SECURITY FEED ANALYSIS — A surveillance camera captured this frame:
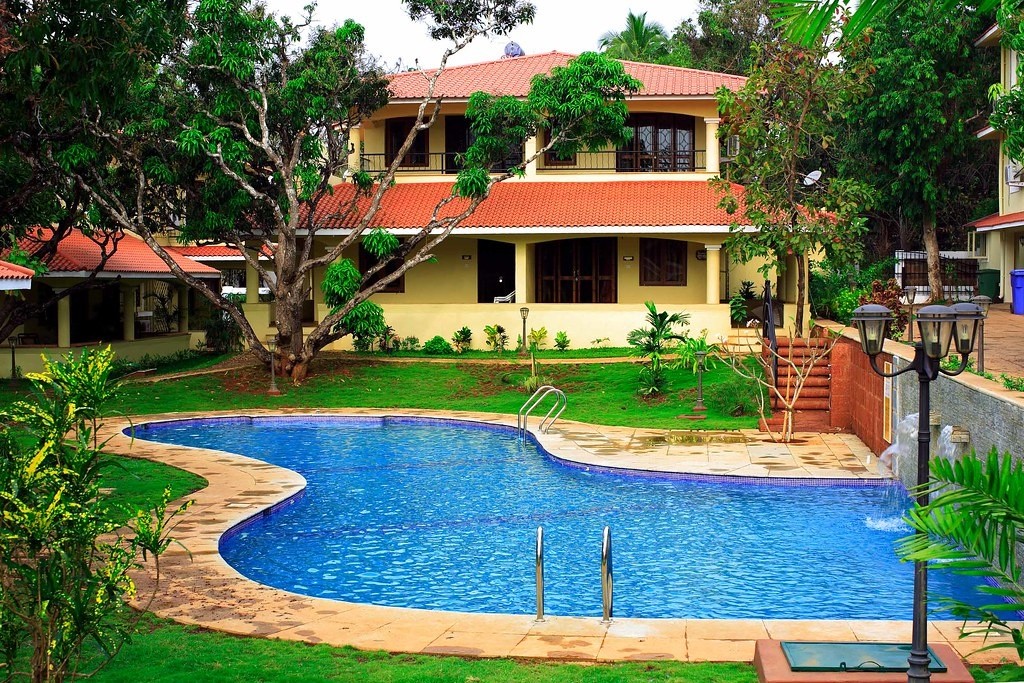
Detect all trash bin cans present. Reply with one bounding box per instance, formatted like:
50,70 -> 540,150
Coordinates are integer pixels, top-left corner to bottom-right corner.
1009,269 -> 1024,314
976,268 -> 1001,302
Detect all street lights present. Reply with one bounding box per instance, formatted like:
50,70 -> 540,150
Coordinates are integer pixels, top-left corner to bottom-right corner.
519,307 -> 529,356
848,298 -> 986,683
693,350 -> 707,410
267,338 -> 281,395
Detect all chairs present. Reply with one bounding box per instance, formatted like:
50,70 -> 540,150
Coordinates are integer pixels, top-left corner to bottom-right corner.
17,333 -> 40,345
494,289 -> 515,304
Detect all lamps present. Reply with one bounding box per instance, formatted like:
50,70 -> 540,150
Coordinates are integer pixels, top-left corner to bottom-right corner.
8,336 -> 21,389
266,339 -> 280,396
903,286 -> 918,347
693,350 -> 708,413
519,306 -> 531,356
972,293 -> 992,377
